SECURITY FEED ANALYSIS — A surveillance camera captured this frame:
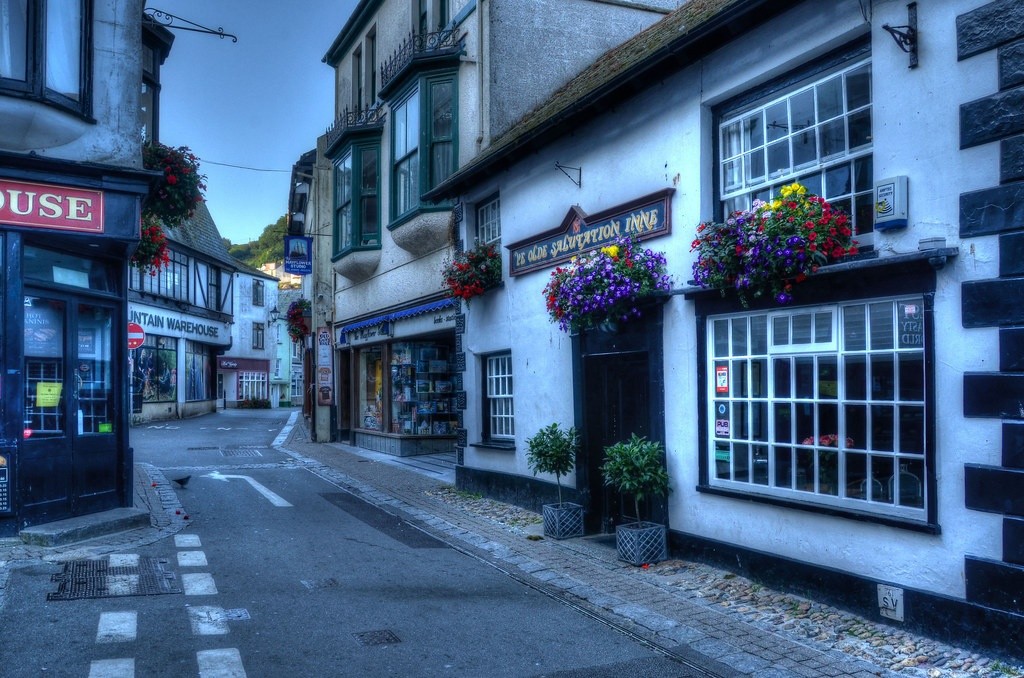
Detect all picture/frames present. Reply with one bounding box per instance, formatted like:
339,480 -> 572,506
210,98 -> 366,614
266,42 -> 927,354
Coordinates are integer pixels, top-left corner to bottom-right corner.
741,401 -> 762,439
741,362 -> 761,398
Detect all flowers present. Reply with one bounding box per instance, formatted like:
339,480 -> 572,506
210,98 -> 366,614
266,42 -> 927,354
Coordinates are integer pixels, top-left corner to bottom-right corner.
802,434 -> 854,470
689,182 -> 861,304
286,299 -> 312,343
541,235 -> 673,333
144,142 -> 207,229
129,219 -> 170,276
442,237 -> 502,300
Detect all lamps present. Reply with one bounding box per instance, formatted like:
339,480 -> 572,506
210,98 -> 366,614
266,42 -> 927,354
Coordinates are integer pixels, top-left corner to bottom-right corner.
268,305 -> 280,328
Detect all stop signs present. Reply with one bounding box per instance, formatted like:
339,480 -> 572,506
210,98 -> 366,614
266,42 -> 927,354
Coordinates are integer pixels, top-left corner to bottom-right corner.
128,321 -> 145,351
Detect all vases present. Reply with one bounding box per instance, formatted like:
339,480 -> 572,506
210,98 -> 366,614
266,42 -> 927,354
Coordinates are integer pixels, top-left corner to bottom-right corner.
302,311 -> 311,330
810,464 -> 835,495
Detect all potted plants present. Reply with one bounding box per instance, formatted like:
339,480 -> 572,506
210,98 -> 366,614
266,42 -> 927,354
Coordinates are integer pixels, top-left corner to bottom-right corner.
523,422 -> 585,540
597,432 -> 674,566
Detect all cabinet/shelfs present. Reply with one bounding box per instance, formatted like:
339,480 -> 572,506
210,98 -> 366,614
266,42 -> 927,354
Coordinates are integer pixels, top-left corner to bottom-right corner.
391,344 -> 457,435
